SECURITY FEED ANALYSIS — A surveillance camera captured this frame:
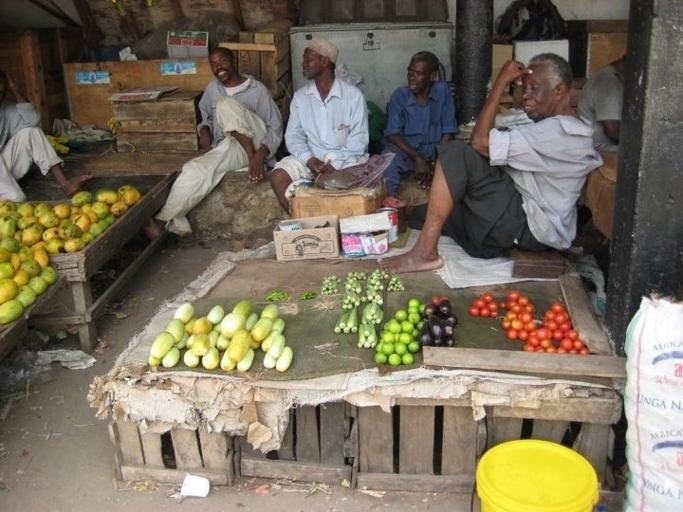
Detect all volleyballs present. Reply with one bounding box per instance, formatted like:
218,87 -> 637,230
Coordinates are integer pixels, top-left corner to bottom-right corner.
419,299 -> 457,346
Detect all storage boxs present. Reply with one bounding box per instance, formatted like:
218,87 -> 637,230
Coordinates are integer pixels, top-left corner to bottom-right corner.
290,176 -> 390,218
580,151 -> 620,241
113,89 -> 207,154
585,19 -> 629,78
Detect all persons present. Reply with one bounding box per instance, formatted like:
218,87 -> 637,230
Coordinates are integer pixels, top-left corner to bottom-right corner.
269,36 -> 371,219
380,51 -> 461,199
576,51 -> 626,154
0,69 -> 94,205
375,51 -> 604,275
142,46 -> 285,240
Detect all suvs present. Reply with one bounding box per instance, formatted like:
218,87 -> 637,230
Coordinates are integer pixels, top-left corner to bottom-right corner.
470,439 -> 605,512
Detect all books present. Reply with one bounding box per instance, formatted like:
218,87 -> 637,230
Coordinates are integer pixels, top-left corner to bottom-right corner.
290,176 -> 390,218
580,151 -> 620,241
113,89 -> 207,154
585,19 -> 629,78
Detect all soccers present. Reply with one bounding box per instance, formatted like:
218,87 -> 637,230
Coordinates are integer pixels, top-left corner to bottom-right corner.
374,298 -> 426,366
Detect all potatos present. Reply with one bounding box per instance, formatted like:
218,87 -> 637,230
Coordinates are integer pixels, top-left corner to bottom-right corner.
148,301 -> 293,372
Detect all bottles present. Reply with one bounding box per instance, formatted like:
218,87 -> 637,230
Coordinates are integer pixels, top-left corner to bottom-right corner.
303,37 -> 339,65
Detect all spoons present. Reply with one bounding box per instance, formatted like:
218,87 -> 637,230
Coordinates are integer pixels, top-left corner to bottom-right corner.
432,295 -> 448,305
469,290 -> 589,354
